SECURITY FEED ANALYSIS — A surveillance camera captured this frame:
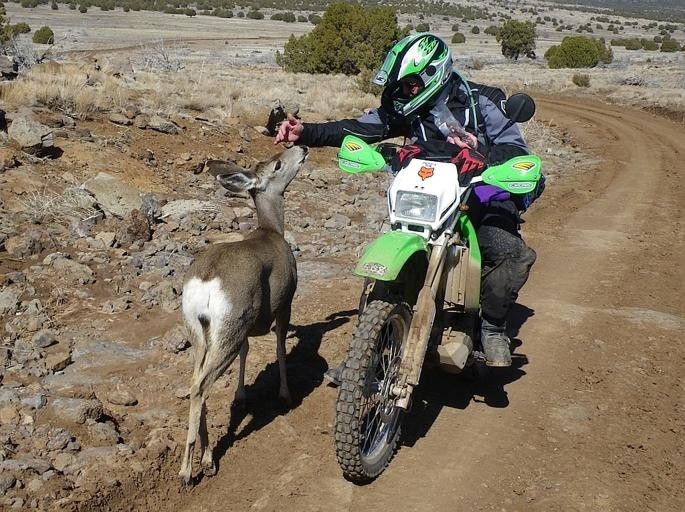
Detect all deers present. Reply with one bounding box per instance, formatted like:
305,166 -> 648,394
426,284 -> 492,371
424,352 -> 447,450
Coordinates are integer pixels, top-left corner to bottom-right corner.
178,145 -> 310,492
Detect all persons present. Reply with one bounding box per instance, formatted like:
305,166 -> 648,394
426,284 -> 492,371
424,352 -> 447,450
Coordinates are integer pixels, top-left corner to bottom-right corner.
272,33 -> 543,386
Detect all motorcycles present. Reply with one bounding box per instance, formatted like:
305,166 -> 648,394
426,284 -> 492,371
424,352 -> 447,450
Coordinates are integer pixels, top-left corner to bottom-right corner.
334,92 -> 538,483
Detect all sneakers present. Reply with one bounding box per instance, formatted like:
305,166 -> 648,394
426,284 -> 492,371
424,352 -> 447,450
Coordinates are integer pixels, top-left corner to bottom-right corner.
479,326 -> 514,368
322,360 -> 349,384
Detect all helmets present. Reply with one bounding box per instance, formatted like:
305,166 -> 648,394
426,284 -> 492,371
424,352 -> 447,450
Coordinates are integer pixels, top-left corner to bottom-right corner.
372,33 -> 461,119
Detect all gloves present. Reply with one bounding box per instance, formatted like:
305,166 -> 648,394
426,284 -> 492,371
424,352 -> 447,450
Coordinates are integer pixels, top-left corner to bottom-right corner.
451,144 -> 491,188
388,140 -> 428,174
